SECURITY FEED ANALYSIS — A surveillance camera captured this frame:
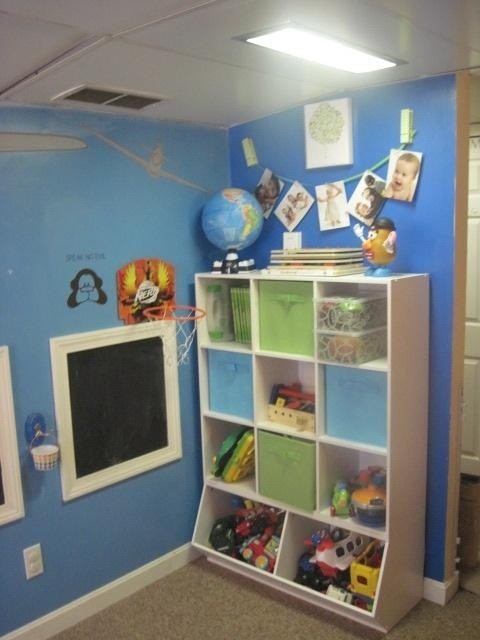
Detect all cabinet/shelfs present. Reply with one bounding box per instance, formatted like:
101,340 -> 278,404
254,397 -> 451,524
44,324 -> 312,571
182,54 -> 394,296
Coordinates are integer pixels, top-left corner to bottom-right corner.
187,268 -> 429,633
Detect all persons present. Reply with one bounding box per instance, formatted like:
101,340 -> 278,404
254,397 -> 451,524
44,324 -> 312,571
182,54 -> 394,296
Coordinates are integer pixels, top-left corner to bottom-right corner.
255,152 -> 420,226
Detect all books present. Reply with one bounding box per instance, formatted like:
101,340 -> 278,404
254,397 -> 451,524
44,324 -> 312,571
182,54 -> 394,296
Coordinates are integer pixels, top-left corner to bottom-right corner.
267,248 -> 365,277
230,283 -> 250,345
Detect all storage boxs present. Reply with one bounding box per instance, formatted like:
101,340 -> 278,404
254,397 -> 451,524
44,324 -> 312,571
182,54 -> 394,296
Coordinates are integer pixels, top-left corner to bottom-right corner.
317,293 -> 384,365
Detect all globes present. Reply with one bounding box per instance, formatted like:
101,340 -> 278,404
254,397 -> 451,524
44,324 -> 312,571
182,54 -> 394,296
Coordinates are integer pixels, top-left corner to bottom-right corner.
200,187 -> 266,274
